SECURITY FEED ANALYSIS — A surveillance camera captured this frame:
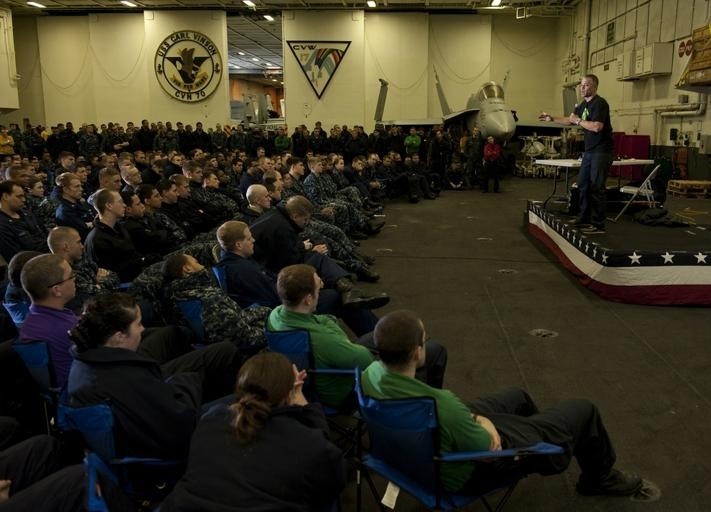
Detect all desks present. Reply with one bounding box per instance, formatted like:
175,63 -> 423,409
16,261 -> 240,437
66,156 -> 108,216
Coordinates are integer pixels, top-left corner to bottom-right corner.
535,157 -> 655,228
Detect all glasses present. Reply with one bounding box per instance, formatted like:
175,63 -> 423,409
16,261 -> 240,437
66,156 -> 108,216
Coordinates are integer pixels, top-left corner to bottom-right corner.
48,272 -> 77,288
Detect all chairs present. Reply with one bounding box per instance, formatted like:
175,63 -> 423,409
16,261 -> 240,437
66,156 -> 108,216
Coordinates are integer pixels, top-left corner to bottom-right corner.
0,238 -> 565,512
607,164 -> 661,222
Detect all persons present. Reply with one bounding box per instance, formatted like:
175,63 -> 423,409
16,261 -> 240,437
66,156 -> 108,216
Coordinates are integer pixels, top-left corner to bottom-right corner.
153,351 -> 343,510
65,291 -> 247,459
1,434 -> 137,510
359,310 -> 644,496
0,119 -> 499,450
539,74 -> 616,235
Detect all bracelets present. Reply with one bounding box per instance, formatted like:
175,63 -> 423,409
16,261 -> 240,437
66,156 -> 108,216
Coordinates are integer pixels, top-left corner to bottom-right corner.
470,410 -> 480,424
577,119 -> 583,125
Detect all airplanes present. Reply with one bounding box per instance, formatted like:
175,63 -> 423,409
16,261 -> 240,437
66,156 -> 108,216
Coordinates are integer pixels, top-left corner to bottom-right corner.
374,64 -> 587,146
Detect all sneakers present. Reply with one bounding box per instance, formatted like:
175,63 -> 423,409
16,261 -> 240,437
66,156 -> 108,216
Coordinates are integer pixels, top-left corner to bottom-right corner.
581,224 -> 606,234
569,219 -> 592,226
576,468 -> 641,496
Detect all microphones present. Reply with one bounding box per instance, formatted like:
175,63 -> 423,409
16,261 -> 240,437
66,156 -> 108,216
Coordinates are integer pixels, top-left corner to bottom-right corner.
575,104 -> 579,115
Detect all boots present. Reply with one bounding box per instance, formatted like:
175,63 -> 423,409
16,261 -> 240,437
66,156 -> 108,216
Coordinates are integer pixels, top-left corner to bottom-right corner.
351,201 -> 386,238
357,254 -> 381,280
336,277 -> 389,310
